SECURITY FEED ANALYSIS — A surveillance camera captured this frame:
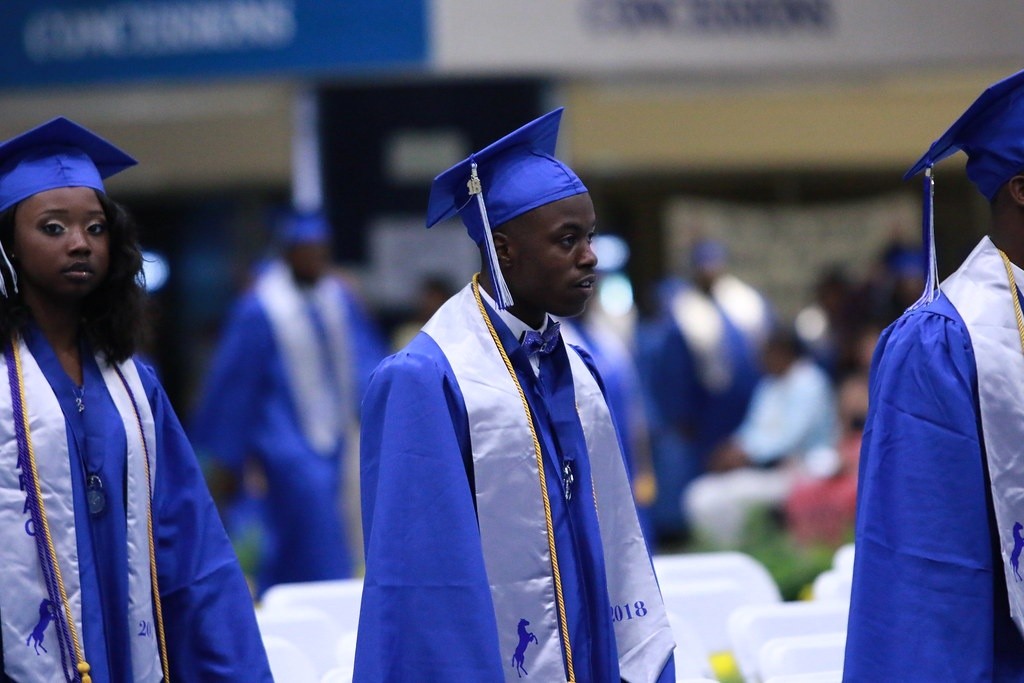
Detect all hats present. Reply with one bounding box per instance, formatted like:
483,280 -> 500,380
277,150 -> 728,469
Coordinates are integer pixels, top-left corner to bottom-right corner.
0,116 -> 139,298
425,106 -> 589,310
903,70 -> 1024,314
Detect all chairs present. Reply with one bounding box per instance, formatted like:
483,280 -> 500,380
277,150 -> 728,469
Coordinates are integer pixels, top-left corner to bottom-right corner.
230,531 -> 861,681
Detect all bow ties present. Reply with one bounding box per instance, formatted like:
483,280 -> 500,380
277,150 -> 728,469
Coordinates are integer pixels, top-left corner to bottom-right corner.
521,322 -> 560,355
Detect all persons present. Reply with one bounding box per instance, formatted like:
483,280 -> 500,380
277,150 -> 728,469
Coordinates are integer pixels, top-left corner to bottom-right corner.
843,71 -> 1024,683
0,117 -> 275,683
186,211 -> 454,601
352,107 -> 677,683
564,234 -> 925,599
